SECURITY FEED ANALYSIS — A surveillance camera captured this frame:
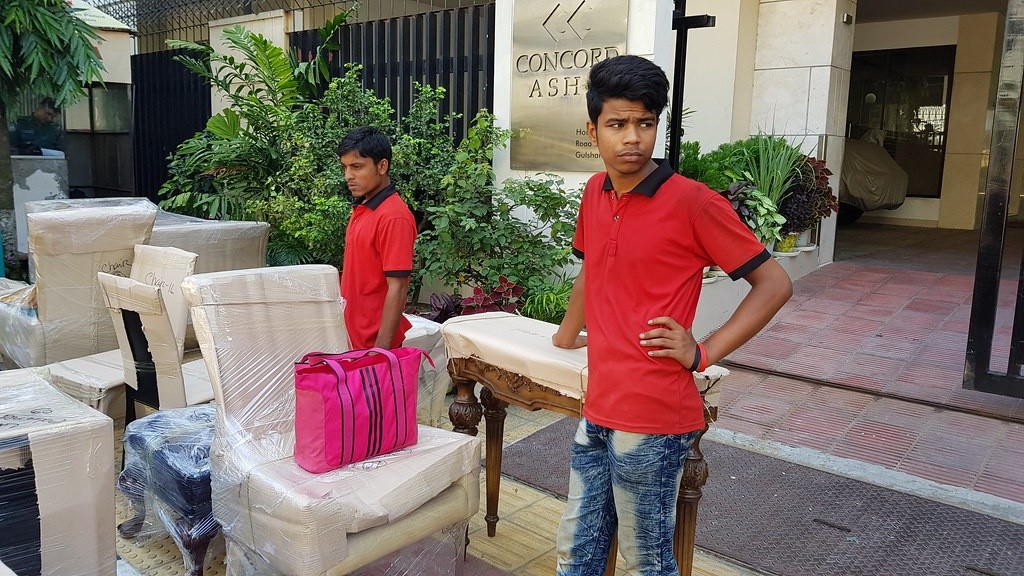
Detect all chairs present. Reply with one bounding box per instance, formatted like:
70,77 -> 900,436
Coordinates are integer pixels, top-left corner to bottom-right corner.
2,202 -> 481,576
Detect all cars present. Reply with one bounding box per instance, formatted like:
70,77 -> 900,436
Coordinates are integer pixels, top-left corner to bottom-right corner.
837,138 -> 910,231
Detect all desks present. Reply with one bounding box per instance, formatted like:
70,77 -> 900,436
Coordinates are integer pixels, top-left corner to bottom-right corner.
440,311 -> 731,576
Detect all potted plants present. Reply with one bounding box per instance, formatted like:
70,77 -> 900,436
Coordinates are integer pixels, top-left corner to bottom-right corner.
725,123 -> 836,257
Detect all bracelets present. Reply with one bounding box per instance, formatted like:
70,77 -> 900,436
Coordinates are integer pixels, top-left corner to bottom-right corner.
688,343 -> 707,373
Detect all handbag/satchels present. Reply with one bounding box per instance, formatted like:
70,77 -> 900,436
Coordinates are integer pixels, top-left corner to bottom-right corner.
293,345 -> 436,474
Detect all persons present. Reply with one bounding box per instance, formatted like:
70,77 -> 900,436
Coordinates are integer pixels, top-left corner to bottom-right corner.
552,54 -> 793,576
336,127 -> 418,358
